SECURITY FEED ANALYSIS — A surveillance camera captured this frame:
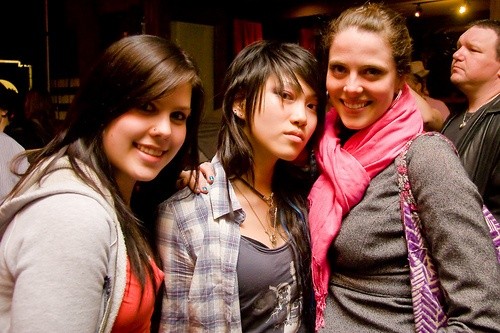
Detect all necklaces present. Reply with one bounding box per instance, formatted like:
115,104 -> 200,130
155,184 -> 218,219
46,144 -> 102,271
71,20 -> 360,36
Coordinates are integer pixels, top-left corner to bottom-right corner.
239,176 -> 280,228
233,182 -> 278,248
459,90 -> 500,128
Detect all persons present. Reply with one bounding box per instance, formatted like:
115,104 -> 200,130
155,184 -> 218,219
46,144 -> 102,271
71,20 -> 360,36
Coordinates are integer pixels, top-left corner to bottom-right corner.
0,0 -> 500,333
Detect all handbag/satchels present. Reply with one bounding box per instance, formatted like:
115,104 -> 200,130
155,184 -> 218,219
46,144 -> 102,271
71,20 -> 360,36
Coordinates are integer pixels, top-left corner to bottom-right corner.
393,130 -> 500,333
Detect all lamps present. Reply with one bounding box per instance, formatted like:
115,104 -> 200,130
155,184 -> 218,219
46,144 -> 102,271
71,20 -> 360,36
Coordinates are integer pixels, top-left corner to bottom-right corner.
414,4 -> 423,17
